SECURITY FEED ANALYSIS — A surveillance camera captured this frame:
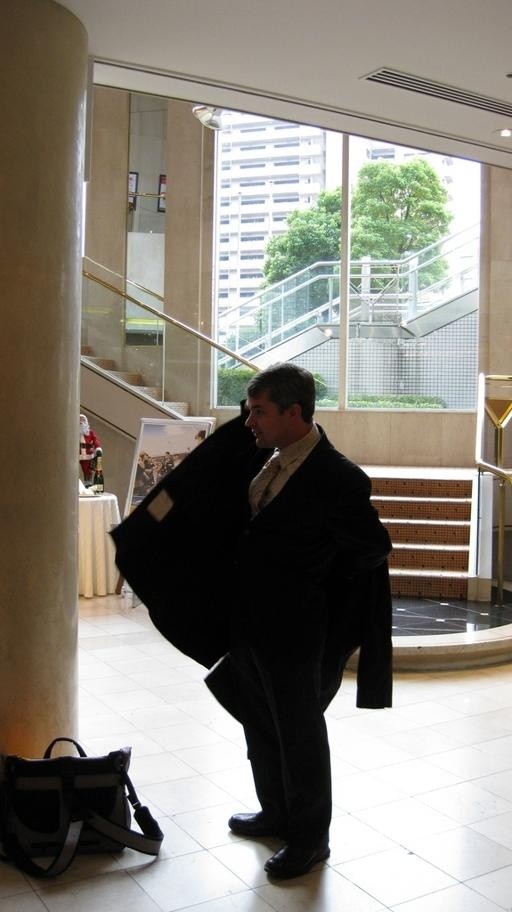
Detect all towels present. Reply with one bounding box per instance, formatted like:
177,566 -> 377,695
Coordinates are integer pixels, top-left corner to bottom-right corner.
78,479 -> 98,495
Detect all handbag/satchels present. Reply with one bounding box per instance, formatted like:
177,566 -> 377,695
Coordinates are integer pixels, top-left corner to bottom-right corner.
0,737 -> 164,880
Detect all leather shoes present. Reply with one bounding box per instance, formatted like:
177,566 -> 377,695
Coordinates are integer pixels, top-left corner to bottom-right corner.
228,810 -> 331,880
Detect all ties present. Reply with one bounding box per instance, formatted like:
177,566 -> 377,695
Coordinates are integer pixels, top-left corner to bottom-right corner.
248,458 -> 281,513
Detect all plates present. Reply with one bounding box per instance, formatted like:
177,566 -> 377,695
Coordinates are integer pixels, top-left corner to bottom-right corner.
79,495 -> 101,498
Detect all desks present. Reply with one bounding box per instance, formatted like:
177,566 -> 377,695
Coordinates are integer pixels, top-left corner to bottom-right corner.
78,491 -> 122,598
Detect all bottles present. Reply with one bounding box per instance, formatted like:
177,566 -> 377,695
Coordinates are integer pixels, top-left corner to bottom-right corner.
94,457 -> 104,493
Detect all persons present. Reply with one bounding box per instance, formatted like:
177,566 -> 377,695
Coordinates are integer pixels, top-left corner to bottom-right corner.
78,412 -> 102,487
207,360 -> 375,882
131,430 -> 205,507
